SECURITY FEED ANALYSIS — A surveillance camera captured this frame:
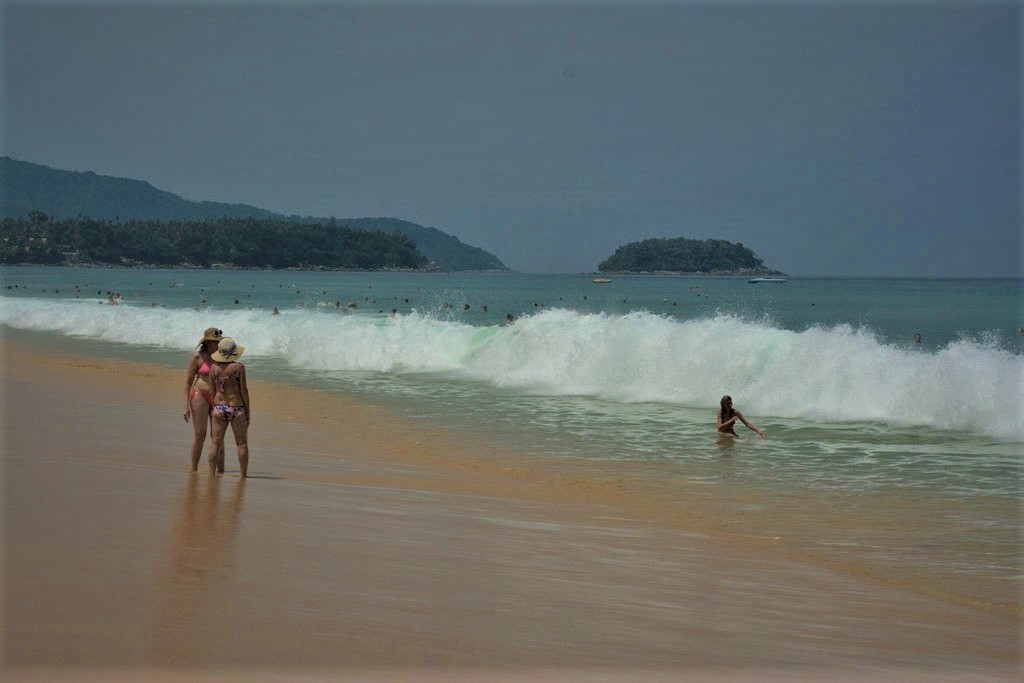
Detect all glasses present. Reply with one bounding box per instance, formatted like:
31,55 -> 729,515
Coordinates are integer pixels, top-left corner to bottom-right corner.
211,330 -> 223,336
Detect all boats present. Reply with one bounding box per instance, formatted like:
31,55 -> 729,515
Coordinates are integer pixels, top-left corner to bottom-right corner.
749,277 -> 787,284
593,279 -> 612,284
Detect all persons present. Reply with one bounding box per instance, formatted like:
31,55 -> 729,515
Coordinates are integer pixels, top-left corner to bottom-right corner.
208,337 -> 250,477
914,333 -> 921,344
183,327 -> 229,474
1,280 -> 679,322
717,395 -> 768,439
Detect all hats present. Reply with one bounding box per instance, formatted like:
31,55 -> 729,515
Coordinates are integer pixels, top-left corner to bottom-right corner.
210,337 -> 244,363
196,327 -> 223,347
